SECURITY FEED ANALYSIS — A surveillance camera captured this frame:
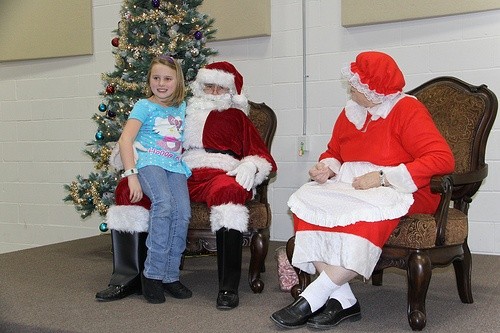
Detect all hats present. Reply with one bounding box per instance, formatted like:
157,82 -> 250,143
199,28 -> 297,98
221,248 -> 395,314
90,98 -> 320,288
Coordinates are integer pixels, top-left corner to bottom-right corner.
340,51 -> 405,104
195,61 -> 244,95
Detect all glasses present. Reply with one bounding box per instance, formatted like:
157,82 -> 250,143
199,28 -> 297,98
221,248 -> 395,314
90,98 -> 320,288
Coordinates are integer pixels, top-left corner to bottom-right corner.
203,88 -> 228,95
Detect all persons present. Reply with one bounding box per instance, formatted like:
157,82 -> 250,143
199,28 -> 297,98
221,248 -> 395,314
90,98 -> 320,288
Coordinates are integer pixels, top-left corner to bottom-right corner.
270,49 -> 456,330
119,55 -> 192,303
93,60 -> 277,309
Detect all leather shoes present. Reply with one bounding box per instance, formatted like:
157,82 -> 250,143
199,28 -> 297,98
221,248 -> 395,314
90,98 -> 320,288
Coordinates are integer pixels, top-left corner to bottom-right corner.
95,276 -> 140,301
307,297 -> 362,330
215,289 -> 239,309
268,296 -> 330,330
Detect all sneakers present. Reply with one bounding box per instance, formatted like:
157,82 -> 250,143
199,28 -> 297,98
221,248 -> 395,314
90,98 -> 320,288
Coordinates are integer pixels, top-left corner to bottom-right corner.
140,271 -> 166,303
163,281 -> 192,299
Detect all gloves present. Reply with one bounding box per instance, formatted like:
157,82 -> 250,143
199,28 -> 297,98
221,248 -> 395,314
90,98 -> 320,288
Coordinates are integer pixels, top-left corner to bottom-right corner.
132,141 -> 146,165
225,161 -> 257,192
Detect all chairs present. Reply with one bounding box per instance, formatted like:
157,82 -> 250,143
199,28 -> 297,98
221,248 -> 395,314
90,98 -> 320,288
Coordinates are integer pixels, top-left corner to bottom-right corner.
120,100 -> 278,293
287,76 -> 498,331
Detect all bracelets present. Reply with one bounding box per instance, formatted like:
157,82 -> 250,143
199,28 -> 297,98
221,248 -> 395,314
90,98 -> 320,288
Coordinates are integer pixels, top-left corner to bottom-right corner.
121,168 -> 138,177
379,171 -> 385,186
